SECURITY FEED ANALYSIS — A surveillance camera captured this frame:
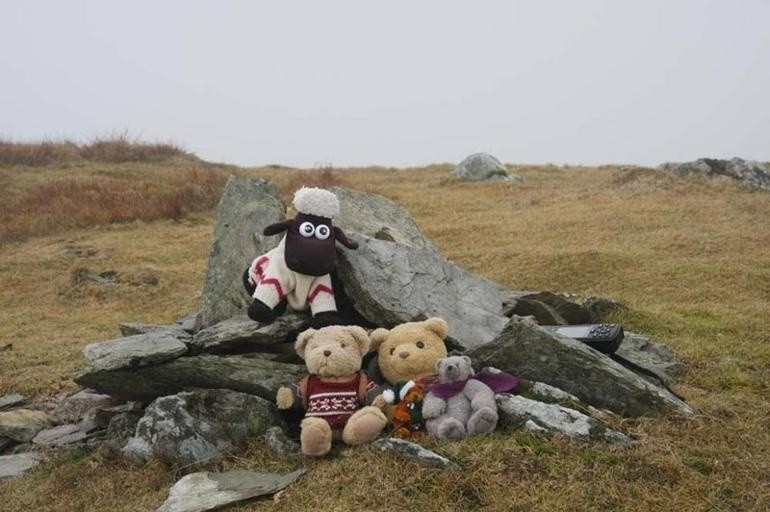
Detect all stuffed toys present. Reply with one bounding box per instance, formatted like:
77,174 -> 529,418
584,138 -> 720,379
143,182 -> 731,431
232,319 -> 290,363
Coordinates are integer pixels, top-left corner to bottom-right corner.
242,188 -> 359,319
421,356 -> 499,439
367,317 -> 448,423
382,379 -> 424,437
276,326 -> 388,456
